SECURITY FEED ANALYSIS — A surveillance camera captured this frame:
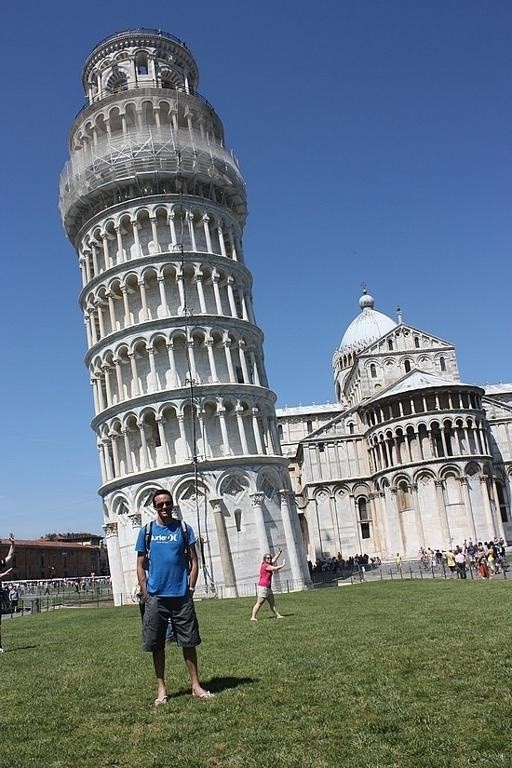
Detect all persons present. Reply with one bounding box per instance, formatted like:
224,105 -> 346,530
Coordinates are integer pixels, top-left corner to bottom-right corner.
135,489 -> 217,707
249,548 -> 287,621
307,535 -> 511,584
2,577 -> 112,613
1,531 -> 17,580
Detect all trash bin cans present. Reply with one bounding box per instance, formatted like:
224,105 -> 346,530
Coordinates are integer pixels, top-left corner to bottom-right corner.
29,598 -> 41,614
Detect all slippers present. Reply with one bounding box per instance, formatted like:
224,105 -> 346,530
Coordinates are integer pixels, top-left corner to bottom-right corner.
191,689 -> 214,698
155,696 -> 169,706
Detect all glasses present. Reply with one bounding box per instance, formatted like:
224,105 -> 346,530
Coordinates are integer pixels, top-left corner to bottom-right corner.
157,500 -> 171,508
